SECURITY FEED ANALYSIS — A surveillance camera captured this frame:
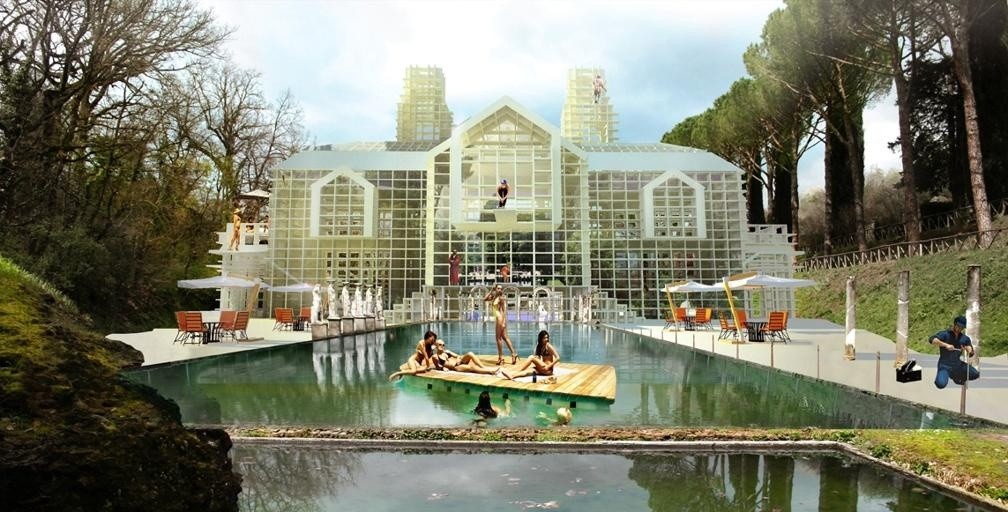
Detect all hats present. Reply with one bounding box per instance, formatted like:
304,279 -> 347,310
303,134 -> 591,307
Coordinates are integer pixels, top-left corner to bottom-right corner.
955,316 -> 966,327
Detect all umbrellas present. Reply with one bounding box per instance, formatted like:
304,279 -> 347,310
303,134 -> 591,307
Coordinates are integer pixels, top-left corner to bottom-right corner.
266,282 -> 315,316
177,270 -> 270,339
240,188 -> 270,222
660,279 -> 726,328
713,271 -> 815,343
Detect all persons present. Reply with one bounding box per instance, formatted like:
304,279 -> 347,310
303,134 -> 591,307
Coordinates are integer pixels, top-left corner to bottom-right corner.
495,179 -> 511,209
501,261 -> 510,283
544,406 -> 572,430
592,75 -> 607,104
482,284 -> 522,365
431,338 -> 500,375
928,315 -> 980,389
501,329 -> 561,381
310,282 -> 383,324
227,207 -> 242,250
388,330 -> 438,382
472,390 -> 501,419
448,249 -> 460,286
502,392 -> 512,417
